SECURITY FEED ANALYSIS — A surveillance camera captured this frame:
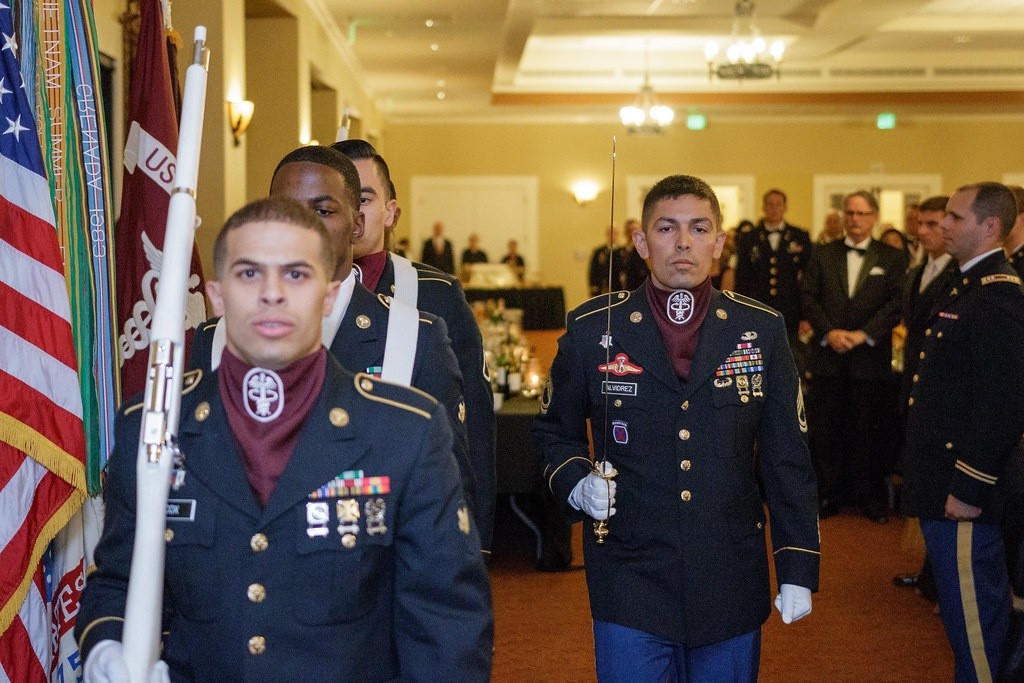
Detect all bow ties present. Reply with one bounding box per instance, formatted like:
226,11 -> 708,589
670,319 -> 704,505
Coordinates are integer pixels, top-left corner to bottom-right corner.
765,229 -> 780,236
844,245 -> 868,257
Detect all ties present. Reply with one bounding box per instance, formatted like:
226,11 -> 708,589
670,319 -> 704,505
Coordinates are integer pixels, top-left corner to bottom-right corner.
919,264 -> 936,291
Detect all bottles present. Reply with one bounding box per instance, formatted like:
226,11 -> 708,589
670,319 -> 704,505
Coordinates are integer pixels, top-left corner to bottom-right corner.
476,297 -> 541,397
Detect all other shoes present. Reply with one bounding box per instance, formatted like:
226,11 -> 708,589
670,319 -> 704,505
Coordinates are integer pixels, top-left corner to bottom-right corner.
864,506 -> 888,525
818,496 -> 837,519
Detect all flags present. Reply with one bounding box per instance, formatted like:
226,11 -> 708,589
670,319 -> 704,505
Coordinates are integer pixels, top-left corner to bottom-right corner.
116,0 -> 207,402
0,0 -> 120,683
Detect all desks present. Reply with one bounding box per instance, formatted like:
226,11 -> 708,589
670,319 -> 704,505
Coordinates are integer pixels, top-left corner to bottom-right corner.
465,285 -> 567,331
491,392 -> 574,571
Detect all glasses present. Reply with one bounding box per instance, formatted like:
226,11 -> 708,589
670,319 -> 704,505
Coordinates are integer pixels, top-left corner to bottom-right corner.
845,210 -> 871,218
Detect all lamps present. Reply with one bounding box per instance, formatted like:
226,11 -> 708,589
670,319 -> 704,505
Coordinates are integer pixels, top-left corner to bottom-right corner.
229,100 -> 254,147
620,73 -> 674,134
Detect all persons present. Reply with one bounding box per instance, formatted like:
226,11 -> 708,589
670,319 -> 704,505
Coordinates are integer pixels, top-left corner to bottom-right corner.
331,138 -> 494,483
463,235 -> 487,264
398,239 -> 412,260
589,220 -> 647,297
906,181 -> 1024,683
894,195 -> 954,597
709,190 -> 922,524
1004,185 -> 1024,611
532,175 -> 822,683
502,240 -> 525,283
73,197 -> 495,682
422,222 -> 456,276
268,145 -> 473,506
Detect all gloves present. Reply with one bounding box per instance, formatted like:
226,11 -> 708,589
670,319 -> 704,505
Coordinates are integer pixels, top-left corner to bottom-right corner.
573,461 -> 616,520
773,583 -> 812,624
82,640 -> 171,683
